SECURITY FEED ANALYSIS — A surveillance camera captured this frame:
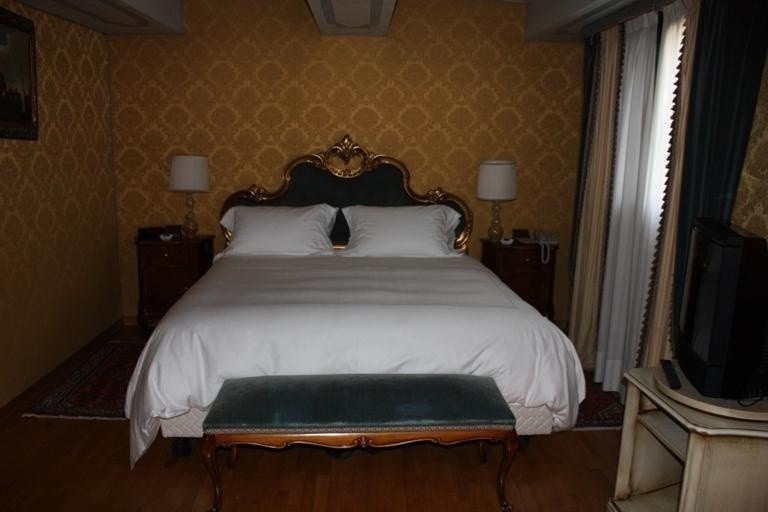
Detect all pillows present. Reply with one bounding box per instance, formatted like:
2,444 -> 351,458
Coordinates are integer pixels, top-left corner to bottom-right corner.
212,202 -> 342,265
339,204 -> 466,259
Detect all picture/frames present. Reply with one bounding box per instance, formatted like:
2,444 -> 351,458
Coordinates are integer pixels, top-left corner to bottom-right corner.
0,7 -> 40,143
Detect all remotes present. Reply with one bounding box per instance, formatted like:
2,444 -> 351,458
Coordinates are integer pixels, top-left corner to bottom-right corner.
660,359 -> 681,389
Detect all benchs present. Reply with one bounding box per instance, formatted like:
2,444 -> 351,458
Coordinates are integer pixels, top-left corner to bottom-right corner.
201,372 -> 519,511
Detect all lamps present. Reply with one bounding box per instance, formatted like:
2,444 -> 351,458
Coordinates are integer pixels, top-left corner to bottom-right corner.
165,155 -> 211,239
476,157 -> 521,240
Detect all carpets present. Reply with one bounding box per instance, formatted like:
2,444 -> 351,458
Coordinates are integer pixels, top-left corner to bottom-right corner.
20,337 -> 625,435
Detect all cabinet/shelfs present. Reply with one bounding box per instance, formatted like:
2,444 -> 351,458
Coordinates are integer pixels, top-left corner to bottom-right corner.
604,357 -> 768,512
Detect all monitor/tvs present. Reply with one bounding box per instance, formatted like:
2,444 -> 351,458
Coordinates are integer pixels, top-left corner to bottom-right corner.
673,217 -> 768,398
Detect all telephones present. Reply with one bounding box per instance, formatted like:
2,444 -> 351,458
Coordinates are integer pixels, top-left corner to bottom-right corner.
534,230 -> 560,245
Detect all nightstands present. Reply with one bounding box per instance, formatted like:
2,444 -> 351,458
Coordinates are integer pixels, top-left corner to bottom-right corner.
477,237 -> 560,323
134,224 -> 217,341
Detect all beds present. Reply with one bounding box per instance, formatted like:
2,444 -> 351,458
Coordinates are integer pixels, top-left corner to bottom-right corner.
122,133 -> 590,472
